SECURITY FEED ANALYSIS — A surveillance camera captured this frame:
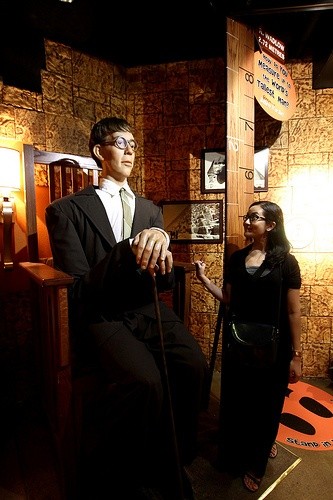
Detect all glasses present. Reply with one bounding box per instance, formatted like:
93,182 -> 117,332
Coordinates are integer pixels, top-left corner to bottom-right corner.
243,214 -> 267,222
99,136 -> 138,152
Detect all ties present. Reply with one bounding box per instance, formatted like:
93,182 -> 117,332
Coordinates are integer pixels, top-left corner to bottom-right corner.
119,188 -> 132,241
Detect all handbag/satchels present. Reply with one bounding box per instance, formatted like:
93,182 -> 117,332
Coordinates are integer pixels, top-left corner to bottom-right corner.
230,322 -> 277,348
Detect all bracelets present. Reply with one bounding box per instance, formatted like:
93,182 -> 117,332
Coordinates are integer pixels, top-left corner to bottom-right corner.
293,350 -> 301,357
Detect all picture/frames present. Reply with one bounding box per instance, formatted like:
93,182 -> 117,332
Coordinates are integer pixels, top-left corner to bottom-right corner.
200,146 -> 269,193
159,199 -> 224,245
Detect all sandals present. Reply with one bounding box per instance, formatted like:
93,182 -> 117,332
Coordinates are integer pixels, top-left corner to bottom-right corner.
269,442 -> 278,458
243,469 -> 261,492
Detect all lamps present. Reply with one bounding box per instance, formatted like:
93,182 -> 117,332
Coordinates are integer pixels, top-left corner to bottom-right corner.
0,145 -> 21,269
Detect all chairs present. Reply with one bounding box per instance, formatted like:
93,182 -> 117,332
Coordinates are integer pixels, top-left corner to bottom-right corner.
18,152 -> 197,500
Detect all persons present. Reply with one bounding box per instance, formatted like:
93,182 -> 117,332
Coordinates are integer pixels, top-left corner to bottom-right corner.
45,118 -> 212,500
192,202 -> 302,491
